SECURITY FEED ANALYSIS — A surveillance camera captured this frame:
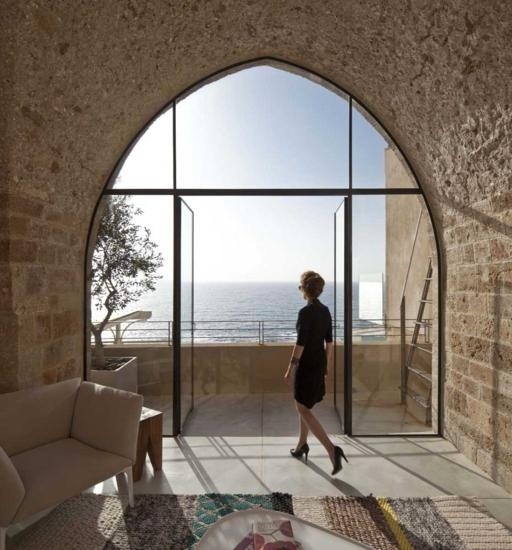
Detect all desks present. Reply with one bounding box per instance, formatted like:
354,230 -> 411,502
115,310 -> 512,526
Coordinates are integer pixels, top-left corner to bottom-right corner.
133,406 -> 164,482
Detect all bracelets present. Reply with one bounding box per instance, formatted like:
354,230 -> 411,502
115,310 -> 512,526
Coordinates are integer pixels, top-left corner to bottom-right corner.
289,357 -> 299,366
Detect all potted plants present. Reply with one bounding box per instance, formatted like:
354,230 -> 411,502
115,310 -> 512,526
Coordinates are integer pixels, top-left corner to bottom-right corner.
91,195 -> 164,395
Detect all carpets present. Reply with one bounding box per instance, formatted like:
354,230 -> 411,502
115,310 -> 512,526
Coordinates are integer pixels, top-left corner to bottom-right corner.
5,493 -> 512,550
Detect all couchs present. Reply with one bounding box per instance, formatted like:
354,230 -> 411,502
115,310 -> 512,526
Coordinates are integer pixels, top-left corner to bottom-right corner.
0,377 -> 144,550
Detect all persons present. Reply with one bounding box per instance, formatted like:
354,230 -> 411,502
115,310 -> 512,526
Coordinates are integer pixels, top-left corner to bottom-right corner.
282,272 -> 351,477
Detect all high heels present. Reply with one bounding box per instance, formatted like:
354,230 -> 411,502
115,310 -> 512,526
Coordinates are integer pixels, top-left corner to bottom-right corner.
328,445 -> 349,478
288,442 -> 310,462
